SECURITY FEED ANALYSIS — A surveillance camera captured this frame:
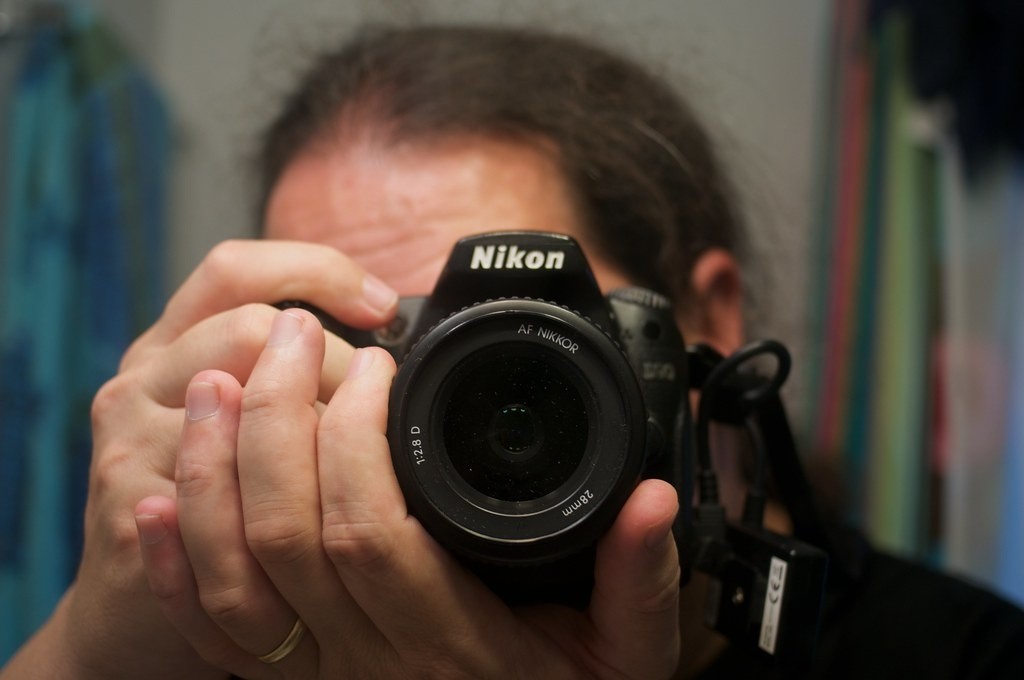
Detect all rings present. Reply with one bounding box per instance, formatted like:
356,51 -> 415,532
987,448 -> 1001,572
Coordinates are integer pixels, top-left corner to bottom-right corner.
257,618 -> 306,664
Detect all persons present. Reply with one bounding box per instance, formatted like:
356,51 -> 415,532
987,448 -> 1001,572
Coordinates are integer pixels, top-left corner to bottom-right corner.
0,21 -> 1024,680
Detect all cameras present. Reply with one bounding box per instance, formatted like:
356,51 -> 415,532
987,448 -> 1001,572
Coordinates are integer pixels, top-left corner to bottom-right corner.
264,227 -> 695,625
